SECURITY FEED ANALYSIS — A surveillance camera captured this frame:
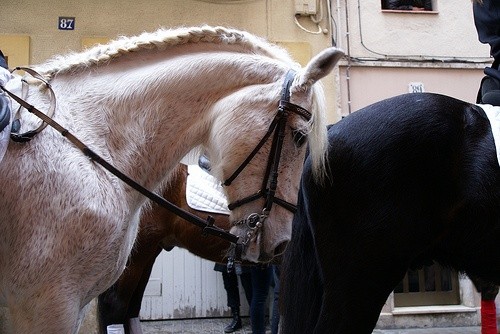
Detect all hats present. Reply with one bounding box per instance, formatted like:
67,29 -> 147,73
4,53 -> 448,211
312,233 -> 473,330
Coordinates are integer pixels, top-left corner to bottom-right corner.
473,0 -> 500,43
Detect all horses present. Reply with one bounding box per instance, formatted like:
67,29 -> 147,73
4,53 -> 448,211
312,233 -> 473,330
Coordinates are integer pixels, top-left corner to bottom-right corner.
0,21 -> 346,334
97,93 -> 500,334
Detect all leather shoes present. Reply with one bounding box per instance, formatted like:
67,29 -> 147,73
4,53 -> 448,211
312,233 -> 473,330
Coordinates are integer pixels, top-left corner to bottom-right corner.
224,318 -> 242,333
